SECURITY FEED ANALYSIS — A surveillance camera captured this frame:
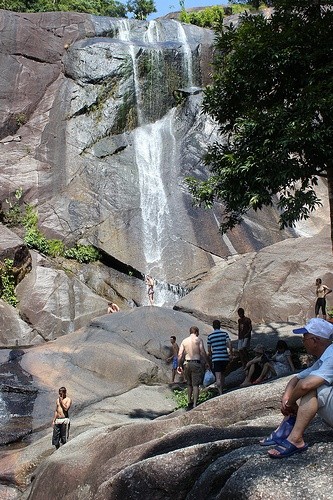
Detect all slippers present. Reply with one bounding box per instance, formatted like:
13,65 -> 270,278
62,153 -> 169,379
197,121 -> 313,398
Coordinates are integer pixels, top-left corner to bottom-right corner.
268,439 -> 309,458
260,430 -> 292,446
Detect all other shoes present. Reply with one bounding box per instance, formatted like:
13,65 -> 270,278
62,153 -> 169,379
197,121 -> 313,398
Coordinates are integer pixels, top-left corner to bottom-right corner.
186,404 -> 194,411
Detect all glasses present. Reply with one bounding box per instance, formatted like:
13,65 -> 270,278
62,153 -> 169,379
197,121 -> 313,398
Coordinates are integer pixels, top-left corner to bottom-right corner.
301,336 -> 319,343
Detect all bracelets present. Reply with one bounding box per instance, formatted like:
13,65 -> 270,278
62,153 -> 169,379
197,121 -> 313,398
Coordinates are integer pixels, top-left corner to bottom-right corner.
285,400 -> 292,409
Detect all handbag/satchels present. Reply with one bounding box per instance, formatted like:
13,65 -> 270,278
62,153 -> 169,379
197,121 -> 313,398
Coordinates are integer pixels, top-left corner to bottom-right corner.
273,412 -> 294,445
203,368 -> 215,387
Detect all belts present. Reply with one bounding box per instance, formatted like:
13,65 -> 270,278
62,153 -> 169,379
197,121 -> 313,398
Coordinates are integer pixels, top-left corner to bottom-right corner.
185,359 -> 201,363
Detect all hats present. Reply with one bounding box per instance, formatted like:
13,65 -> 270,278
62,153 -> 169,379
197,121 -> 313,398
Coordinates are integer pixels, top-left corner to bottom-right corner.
292,318 -> 333,341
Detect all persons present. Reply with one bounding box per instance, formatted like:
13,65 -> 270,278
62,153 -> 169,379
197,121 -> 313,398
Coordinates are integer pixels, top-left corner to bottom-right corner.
315,278 -> 333,319
237,307 -> 252,359
259,317 -> 333,458
207,320 -> 232,395
240,344 -> 272,387
107,301 -> 119,313
146,275 -> 154,306
252,340 -> 295,384
176,326 -> 210,410
51,387 -> 72,451
166,335 -> 184,382
